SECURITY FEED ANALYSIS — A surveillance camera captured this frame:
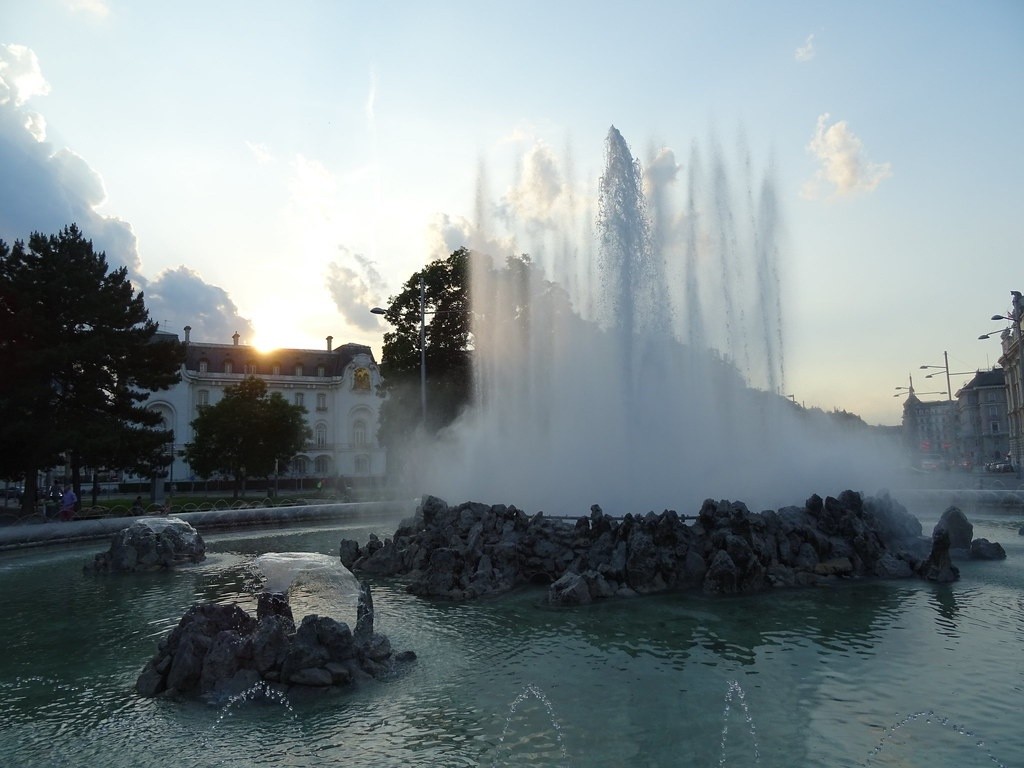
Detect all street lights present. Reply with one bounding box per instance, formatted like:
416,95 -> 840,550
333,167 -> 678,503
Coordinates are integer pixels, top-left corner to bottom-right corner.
919,351 -> 956,442
370,277 -> 473,425
977,297 -> 1024,402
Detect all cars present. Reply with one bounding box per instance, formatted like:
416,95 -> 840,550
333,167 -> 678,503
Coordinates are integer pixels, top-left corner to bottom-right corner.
37,485 -> 65,500
0,486 -> 24,499
921,453 -> 974,473
984,458 -> 1013,473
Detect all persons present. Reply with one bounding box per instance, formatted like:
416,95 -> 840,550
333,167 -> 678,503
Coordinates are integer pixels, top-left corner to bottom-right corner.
134,496 -> 143,510
48,480 -> 78,518
337,475 -> 347,496
317,481 -> 322,491
172,483 -> 177,497
